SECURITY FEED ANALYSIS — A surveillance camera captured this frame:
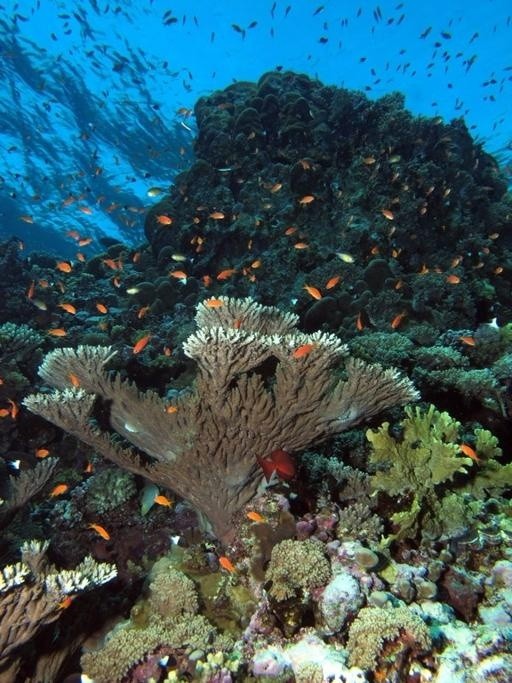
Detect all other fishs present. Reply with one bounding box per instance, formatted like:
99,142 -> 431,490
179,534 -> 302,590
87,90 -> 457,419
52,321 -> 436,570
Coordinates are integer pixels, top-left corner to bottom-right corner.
2,0 -> 512,683
